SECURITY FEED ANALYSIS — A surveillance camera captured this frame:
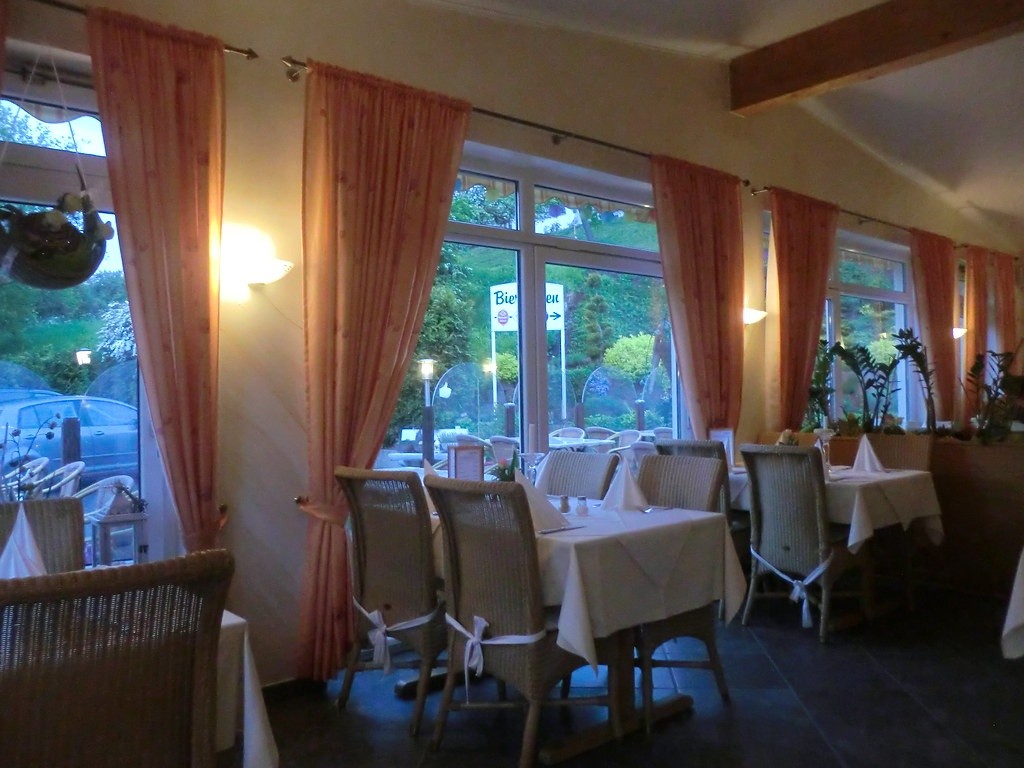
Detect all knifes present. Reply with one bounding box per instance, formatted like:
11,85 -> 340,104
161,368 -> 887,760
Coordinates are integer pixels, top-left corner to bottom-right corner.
538,525 -> 588,534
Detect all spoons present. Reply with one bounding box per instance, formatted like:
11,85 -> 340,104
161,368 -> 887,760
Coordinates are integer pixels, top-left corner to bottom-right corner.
643,506 -> 673,513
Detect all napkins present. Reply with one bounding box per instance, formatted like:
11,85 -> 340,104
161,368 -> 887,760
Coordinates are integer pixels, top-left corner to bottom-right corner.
601,455 -> 649,511
849,432 -> 887,471
0,503 -> 47,578
515,451 -> 570,531
424,459 -> 437,512
775,430 -> 831,481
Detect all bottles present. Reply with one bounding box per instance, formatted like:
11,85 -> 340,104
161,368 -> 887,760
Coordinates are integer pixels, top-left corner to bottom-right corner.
559,495 -> 568,513
576,496 -> 588,515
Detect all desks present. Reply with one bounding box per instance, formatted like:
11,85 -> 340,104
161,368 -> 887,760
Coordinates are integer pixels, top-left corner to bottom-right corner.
486,436 -> 615,451
364,494 -> 749,764
726,464 -> 946,627
615,430 -> 656,436
389,453 -> 448,462
0,584 -> 281,768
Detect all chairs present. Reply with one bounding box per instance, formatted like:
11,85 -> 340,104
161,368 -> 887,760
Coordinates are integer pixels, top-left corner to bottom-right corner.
655,441 -> 773,619
424,474 -> 624,768
855,434 -> 948,612
0,457 -> 139,560
494,451 -> 620,698
335,464 -> 508,737
0,496 -> 85,578
739,443 -> 878,643
0,546 -> 235,768
758,431 -> 817,446
388,422 -> 675,472
561,452 -> 729,737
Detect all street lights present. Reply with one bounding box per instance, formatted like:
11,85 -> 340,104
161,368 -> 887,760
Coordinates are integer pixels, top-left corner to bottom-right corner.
418,357 -> 438,467
74,346 -> 92,397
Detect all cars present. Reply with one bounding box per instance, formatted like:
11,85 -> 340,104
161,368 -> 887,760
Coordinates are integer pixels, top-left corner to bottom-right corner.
0,388 -> 64,404
0,395 -> 138,481
